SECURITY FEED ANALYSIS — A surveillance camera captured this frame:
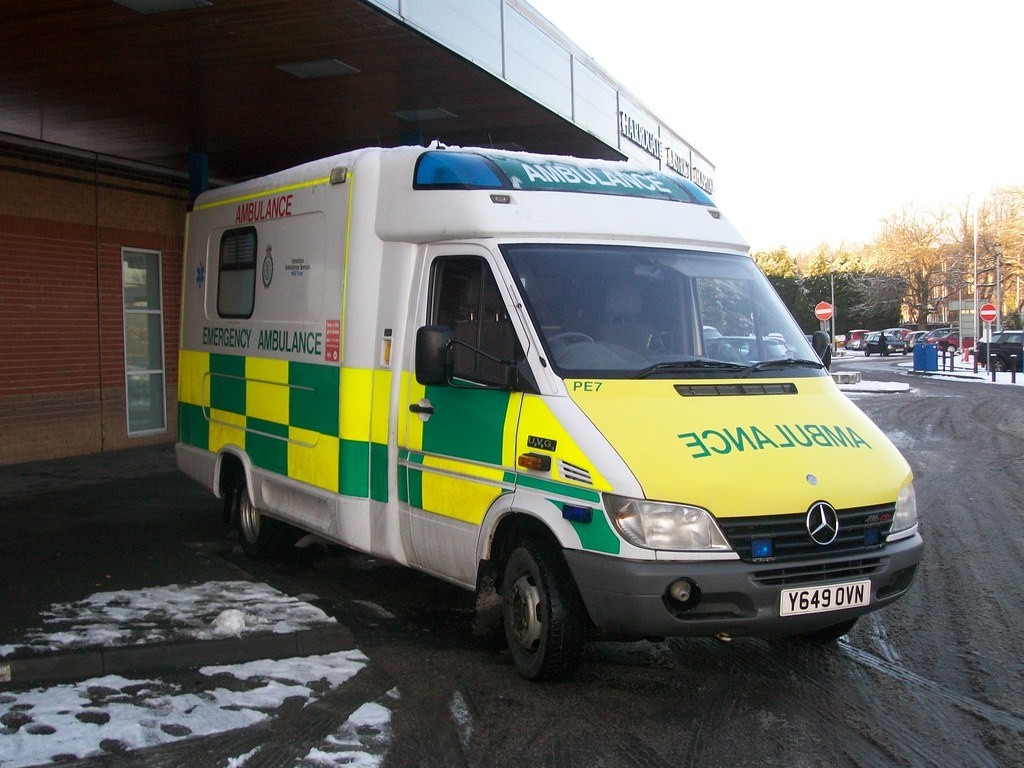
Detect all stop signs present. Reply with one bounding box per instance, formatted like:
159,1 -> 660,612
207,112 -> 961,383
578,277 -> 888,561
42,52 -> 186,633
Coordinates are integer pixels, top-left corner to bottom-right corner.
814,301 -> 833,321
980,303 -> 997,322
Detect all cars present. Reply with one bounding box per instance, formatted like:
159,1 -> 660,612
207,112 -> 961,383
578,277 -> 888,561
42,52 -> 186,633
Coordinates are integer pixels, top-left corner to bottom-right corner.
934,331 -> 974,353
701,327 -> 731,349
862,334 -> 908,357
975,331 -> 1024,372
844,328 -> 930,353
705,337 -> 793,366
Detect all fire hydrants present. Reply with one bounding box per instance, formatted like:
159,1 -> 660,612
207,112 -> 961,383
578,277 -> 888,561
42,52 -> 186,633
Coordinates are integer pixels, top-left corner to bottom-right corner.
965,348 -> 970,361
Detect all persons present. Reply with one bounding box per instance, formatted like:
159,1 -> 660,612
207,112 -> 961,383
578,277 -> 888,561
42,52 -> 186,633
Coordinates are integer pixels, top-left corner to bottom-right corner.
878,331 -> 889,356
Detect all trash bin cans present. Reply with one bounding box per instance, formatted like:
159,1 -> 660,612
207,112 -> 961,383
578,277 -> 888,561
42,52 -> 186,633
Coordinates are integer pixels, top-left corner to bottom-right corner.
913,341 -> 939,373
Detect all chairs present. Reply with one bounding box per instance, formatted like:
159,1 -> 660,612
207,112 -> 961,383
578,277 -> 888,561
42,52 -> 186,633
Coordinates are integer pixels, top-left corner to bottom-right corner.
452,273 -> 518,383
593,280 -> 665,352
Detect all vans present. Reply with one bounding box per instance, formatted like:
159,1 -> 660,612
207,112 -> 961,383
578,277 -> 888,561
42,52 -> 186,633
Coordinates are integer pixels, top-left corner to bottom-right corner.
923,328 -> 959,344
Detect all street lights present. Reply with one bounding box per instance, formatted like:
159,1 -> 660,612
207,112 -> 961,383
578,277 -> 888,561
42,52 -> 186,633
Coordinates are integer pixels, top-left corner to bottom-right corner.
994,242 -> 1001,331
829,264 -> 835,352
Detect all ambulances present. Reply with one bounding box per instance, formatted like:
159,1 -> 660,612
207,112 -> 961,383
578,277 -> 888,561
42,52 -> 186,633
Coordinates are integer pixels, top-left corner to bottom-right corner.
176,139 -> 924,682
